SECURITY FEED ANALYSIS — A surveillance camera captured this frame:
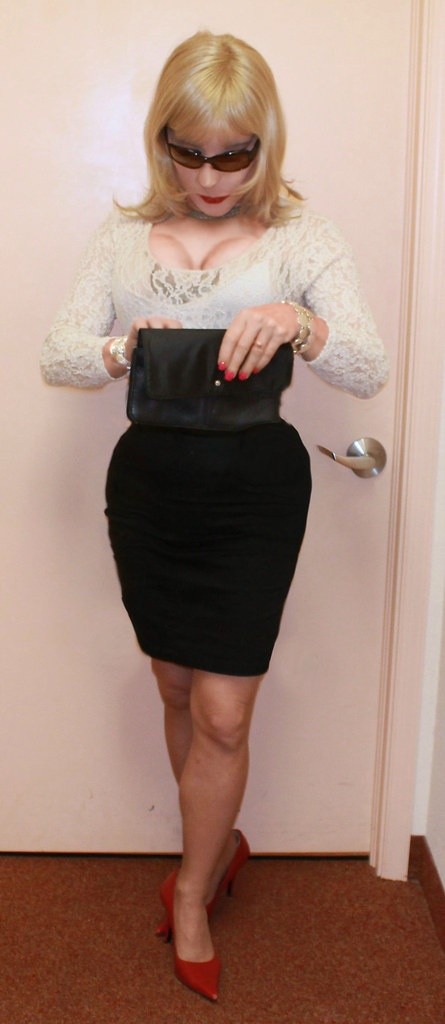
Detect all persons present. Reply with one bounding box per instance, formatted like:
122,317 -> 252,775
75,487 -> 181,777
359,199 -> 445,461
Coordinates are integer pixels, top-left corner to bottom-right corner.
36,30 -> 392,1002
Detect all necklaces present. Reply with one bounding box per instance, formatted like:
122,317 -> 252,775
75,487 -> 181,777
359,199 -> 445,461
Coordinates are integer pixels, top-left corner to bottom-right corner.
185,206 -> 243,221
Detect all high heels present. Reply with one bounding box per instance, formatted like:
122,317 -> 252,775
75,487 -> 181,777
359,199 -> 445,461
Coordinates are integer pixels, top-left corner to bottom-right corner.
156,829 -> 250,934
159,867 -> 221,1002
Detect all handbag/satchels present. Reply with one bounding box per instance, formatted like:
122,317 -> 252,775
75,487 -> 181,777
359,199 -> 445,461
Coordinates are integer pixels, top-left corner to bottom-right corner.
127,328 -> 293,432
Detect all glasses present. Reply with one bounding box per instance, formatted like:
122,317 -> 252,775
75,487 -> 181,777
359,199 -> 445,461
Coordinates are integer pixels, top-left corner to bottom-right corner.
165,127 -> 260,173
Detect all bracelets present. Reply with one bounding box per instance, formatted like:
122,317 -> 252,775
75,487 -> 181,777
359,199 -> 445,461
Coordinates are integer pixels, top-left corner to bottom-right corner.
110,336 -> 132,371
282,300 -> 314,355
